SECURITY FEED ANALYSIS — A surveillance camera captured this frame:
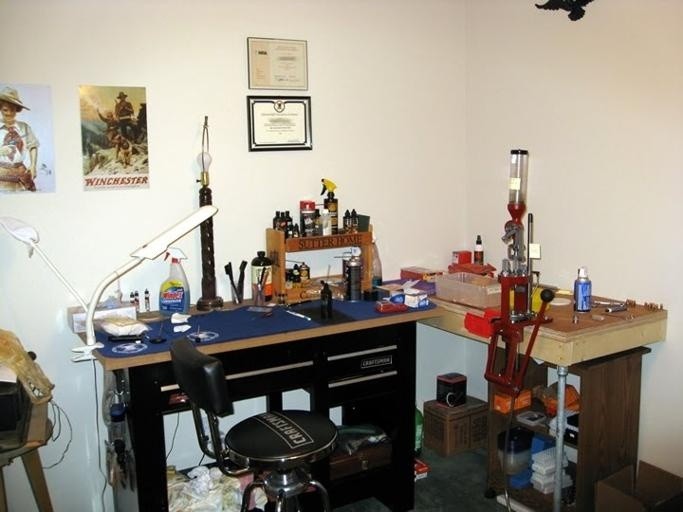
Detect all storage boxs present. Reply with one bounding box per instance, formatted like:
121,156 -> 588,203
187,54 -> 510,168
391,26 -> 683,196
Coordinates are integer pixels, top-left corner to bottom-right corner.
327,439 -> 394,481
422,394 -> 487,458
592,460 -> 683,512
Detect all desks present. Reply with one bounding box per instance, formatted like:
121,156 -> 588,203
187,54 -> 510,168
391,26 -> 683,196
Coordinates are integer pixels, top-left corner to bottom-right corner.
66,291 -> 445,512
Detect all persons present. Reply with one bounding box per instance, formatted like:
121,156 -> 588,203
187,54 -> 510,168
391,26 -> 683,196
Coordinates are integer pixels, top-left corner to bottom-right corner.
112,135 -> 132,168
0,85 -> 38,193
97,108 -> 121,146
114,91 -> 137,142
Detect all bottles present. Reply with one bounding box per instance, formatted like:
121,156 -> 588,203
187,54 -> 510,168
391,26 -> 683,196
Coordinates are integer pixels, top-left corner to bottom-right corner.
473,234 -> 484,265
320,283 -> 332,320
272,210 -> 299,239
371,239 -> 383,288
342,209 -> 358,235
284,261 -> 310,282
320,197 -> 331,236
572,265 -> 592,313
343,255 -> 361,301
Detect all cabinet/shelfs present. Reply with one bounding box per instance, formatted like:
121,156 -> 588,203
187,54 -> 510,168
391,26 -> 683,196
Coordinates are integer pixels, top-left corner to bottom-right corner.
487,344 -> 640,511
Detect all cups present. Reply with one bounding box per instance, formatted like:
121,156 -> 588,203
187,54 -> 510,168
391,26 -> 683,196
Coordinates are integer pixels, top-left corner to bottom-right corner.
251,286 -> 265,308
230,281 -> 243,305
300,211 -> 315,237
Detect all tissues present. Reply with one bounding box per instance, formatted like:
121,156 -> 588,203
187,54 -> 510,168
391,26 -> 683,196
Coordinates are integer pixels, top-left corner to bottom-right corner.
378,280 -> 430,308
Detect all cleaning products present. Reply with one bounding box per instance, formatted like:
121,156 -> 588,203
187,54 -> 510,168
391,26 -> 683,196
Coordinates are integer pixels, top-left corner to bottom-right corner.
573,267 -> 590,312
159,248 -> 190,316
474,235 -> 484,266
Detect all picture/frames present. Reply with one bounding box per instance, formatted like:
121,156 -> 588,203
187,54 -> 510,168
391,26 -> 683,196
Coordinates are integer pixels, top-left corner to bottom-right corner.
247,37 -> 312,152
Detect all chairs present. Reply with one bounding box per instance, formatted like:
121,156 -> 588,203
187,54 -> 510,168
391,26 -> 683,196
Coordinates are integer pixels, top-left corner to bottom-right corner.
168,338 -> 340,511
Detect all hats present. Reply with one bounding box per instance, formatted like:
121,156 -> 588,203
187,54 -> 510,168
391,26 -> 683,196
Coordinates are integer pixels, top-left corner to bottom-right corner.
117,92 -> 127,99
1,87 -> 30,111
